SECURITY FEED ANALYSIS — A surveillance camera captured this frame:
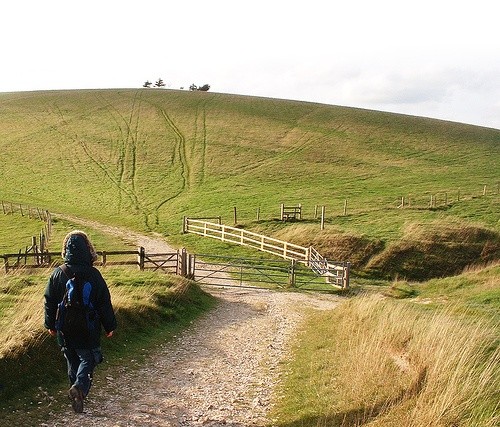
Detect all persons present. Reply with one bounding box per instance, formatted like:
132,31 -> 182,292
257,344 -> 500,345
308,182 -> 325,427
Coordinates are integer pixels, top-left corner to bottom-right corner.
44,230 -> 117,414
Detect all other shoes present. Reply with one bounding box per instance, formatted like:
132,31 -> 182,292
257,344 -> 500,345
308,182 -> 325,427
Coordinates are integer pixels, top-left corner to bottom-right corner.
69,384 -> 84,414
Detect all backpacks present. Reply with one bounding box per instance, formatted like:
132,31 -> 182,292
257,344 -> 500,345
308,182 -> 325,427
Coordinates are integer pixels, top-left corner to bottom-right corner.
59,263 -> 99,334
